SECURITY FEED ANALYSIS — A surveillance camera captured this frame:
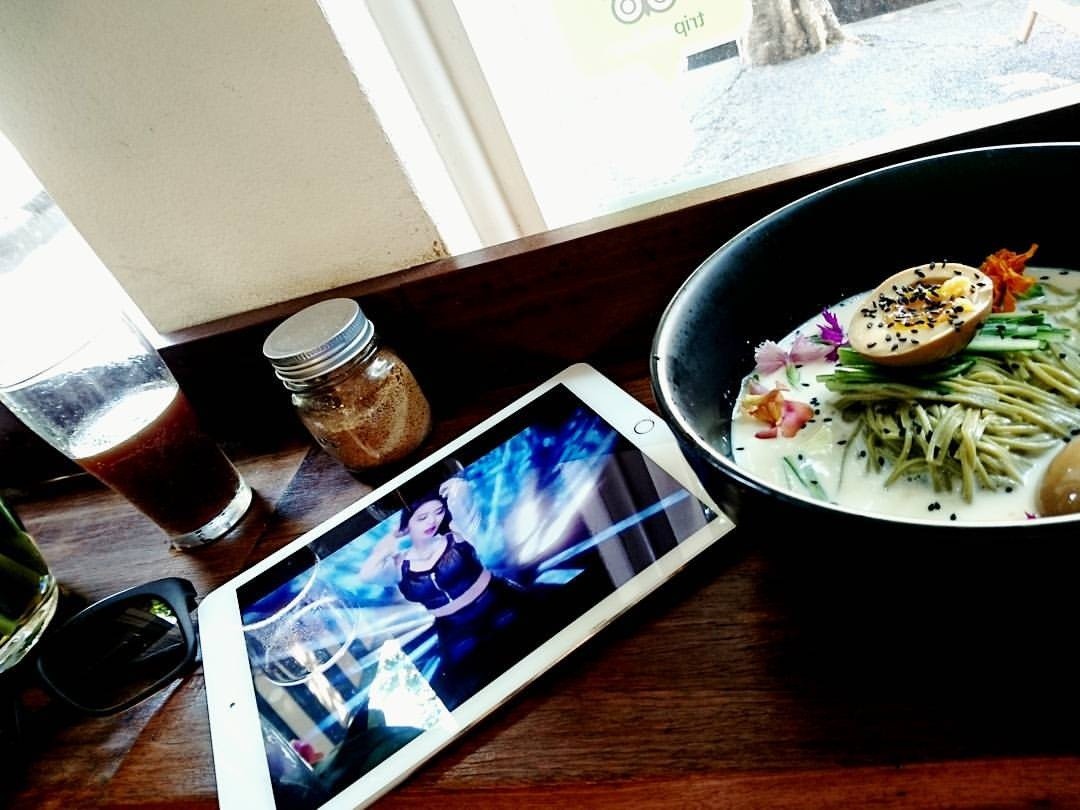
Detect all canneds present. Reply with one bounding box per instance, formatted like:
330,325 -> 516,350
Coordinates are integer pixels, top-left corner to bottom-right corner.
263,298 -> 431,472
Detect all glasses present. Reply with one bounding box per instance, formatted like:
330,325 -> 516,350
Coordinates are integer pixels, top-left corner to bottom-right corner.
0,573 -> 197,758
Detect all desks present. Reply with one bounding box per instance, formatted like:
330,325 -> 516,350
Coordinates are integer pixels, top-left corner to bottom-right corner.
0,86 -> 1080,810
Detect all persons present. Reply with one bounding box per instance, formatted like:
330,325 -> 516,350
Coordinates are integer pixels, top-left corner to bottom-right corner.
368,475 -> 586,717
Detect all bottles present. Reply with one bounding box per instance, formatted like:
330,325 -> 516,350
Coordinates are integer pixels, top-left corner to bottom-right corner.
262,298 -> 434,490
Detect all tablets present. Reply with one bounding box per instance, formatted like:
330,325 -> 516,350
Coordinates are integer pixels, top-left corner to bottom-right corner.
196,361 -> 747,810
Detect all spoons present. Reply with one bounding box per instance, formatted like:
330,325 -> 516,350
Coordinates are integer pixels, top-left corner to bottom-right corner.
1033,440 -> 1080,519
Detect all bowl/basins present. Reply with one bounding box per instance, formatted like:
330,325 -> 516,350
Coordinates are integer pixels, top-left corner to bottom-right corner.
648,142 -> 1079,527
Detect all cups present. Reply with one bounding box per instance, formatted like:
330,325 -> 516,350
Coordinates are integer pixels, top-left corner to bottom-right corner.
1,305 -> 253,552
0,502 -> 60,676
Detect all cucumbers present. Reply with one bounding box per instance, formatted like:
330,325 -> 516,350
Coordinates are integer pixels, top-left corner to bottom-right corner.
816,310 -> 1071,389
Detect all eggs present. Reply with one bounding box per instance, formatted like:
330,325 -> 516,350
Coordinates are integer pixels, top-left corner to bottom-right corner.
847,262 -> 994,368
1036,434 -> 1080,517
